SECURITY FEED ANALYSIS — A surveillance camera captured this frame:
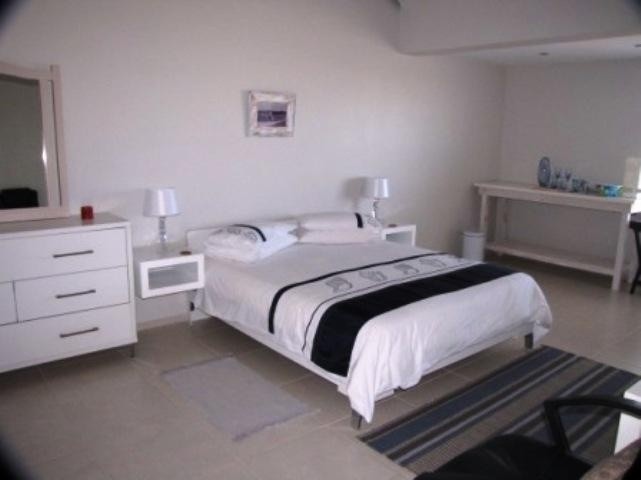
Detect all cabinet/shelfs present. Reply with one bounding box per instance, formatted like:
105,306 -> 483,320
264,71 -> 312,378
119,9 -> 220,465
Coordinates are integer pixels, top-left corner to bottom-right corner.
0,211 -> 138,373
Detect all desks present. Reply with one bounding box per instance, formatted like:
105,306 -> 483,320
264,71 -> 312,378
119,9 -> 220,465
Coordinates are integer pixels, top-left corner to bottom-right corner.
474,179 -> 640,290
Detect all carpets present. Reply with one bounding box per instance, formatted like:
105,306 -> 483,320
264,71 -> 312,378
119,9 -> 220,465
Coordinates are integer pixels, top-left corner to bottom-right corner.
356,344 -> 641,475
161,354 -> 313,441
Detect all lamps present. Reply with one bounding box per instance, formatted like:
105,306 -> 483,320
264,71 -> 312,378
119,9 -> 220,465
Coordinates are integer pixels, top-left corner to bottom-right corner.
363,178 -> 390,219
142,187 -> 180,255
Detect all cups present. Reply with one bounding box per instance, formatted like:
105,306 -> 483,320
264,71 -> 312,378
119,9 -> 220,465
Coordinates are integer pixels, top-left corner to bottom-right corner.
81,207 -> 93,219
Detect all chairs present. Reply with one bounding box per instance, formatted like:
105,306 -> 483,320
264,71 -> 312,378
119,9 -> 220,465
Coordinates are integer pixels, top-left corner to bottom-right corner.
413,395 -> 641,479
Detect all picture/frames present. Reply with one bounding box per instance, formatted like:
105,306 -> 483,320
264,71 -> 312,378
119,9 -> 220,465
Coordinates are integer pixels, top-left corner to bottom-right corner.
247,90 -> 295,138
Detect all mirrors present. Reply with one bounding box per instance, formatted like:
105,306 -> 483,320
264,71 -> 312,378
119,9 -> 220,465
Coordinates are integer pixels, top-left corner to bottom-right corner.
1,62 -> 71,221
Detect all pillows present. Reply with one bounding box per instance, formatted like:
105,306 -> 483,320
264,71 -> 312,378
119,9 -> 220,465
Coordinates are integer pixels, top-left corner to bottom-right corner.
200,236 -> 298,263
298,231 -> 374,244
301,212 -> 368,230
206,224 -> 299,248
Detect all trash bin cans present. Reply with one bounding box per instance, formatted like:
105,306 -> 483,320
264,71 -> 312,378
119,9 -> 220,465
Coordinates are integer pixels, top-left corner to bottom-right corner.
461,230 -> 487,261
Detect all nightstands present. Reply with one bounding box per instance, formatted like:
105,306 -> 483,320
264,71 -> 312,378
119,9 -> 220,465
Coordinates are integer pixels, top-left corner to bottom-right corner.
133,243 -> 206,299
381,221 -> 417,246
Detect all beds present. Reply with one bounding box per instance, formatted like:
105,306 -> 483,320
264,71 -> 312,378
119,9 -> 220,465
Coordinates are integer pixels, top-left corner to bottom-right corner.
187,212 -> 551,431
